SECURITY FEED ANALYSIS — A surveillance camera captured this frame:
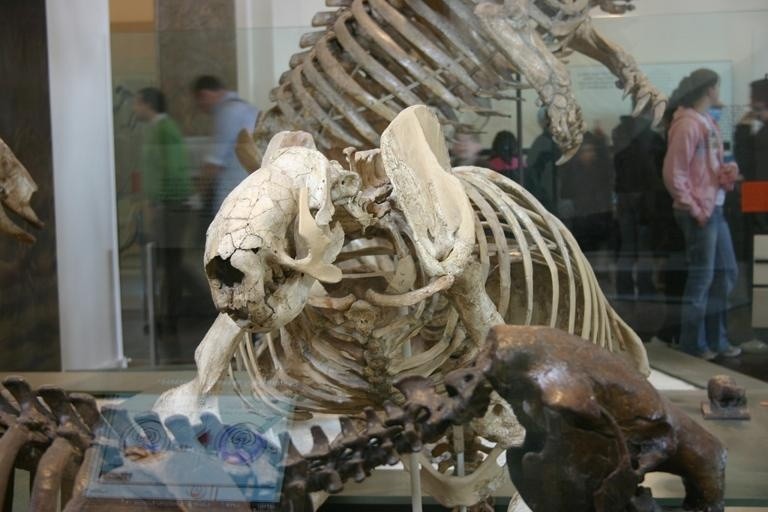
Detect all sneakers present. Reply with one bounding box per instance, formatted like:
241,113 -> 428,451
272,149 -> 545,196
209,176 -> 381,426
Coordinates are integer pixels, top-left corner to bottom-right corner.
693,350 -> 718,361
737,338 -> 767,354
720,345 -> 741,358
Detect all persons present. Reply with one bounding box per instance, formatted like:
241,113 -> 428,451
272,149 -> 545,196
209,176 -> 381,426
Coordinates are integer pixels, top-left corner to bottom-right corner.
130,83 -> 204,346
182,77 -> 265,221
449,66 -> 766,361
